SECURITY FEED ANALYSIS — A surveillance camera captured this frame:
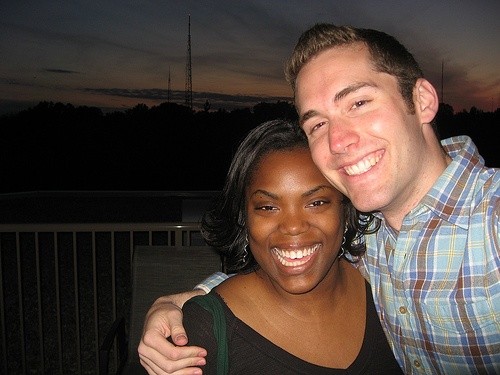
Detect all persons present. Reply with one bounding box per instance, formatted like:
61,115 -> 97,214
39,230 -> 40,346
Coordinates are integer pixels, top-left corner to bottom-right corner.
164,118 -> 405,375
136,21 -> 500,375
